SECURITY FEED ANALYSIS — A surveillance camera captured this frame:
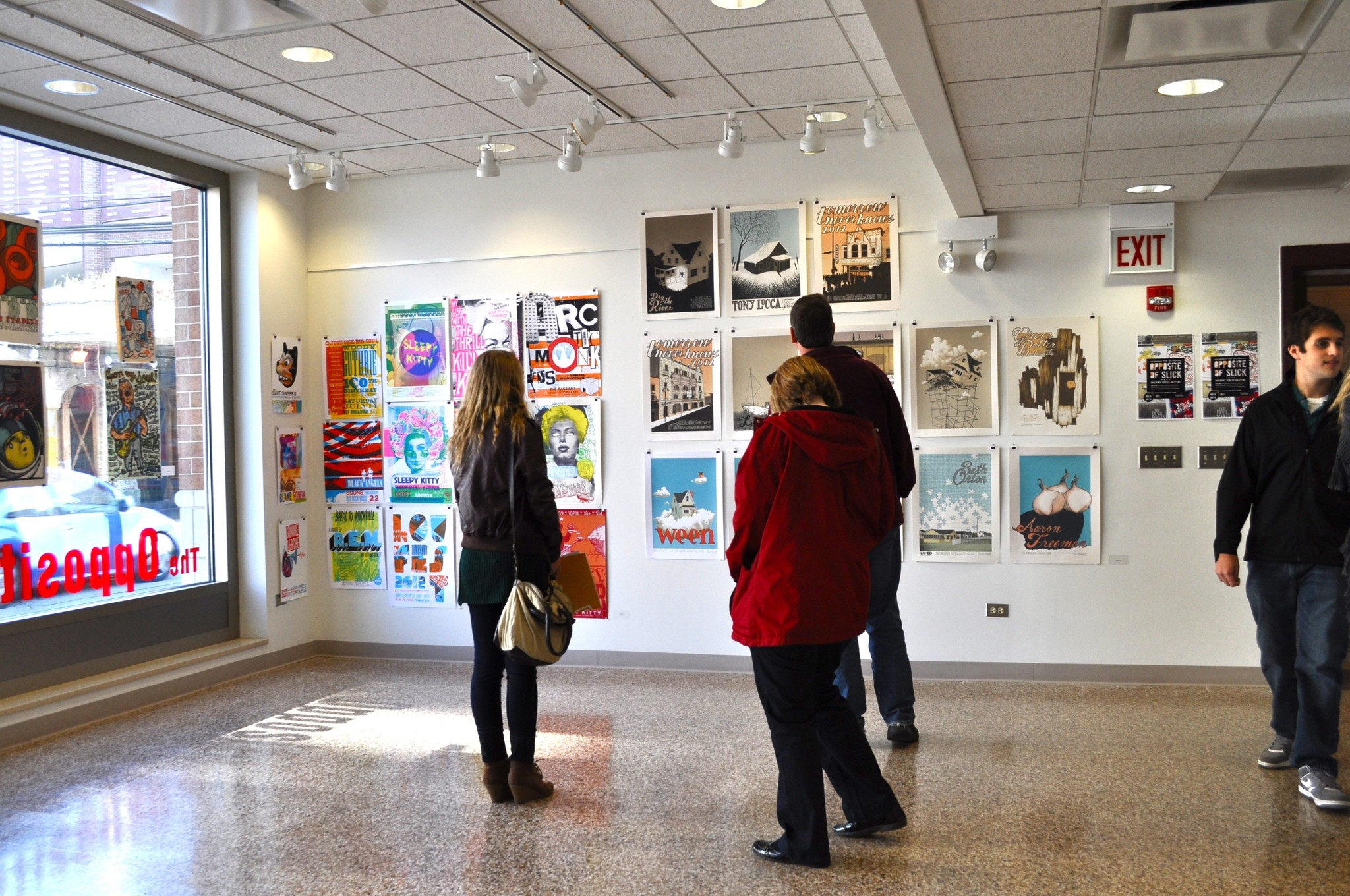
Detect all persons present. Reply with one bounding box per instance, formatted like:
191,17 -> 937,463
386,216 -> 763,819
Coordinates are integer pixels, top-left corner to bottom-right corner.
450,350 -> 563,806
1212,306 -> 1350,809
767,295 -> 920,743
725,358 -> 907,871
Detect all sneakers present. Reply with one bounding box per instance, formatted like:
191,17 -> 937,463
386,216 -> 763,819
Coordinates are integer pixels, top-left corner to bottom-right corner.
1258,735 -> 1295,767
1297,764 -> 1349,807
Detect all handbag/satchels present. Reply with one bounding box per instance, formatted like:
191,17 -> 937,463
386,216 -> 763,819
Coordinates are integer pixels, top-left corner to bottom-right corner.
493,579 -> 576,667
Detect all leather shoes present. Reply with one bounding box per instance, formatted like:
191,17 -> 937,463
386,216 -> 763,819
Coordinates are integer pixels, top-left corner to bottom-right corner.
752,840 -> 831,868
832,816 -> 907,836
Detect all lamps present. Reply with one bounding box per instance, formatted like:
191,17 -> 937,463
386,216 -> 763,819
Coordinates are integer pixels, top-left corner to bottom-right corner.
288,147 -> 313,190
558,127 -> 582,173
325,151 -> 350,193
476,136 -> 500,176
799,104 -> 826,155
718,112 -> 743,158
495,52 -> 549,108
936,215 -> 1000,273
862,99 -> 891,149
571,95 -> 606,145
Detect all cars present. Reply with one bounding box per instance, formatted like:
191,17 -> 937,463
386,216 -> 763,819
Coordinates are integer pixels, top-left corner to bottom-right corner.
0,470 -> 179,605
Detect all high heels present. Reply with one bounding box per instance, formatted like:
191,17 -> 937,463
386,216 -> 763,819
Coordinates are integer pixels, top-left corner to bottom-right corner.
508,759 -> 554,803
482,762 -> 513,802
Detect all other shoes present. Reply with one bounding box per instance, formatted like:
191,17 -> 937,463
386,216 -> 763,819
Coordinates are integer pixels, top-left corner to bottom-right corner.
888,721 -> 919,740
